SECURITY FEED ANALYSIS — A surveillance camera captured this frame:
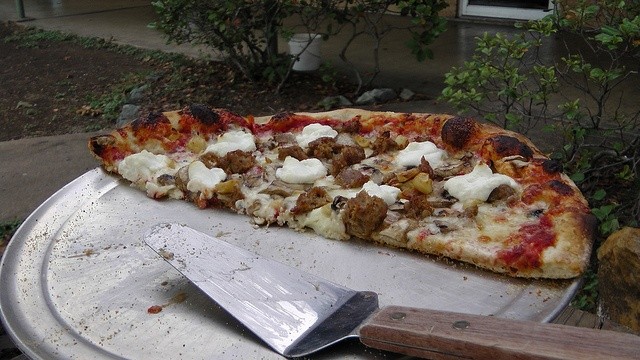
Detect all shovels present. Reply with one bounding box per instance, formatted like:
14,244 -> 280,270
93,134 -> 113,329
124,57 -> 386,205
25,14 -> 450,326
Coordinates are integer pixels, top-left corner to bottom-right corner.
143,222 -> 640,360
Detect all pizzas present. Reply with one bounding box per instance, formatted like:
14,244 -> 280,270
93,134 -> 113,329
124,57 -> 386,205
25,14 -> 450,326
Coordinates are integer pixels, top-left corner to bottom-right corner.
89,107 -> 597,280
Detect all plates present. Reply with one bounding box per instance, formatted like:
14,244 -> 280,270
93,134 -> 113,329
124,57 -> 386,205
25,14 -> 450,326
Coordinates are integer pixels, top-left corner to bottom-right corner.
0,166 -> 583,360
